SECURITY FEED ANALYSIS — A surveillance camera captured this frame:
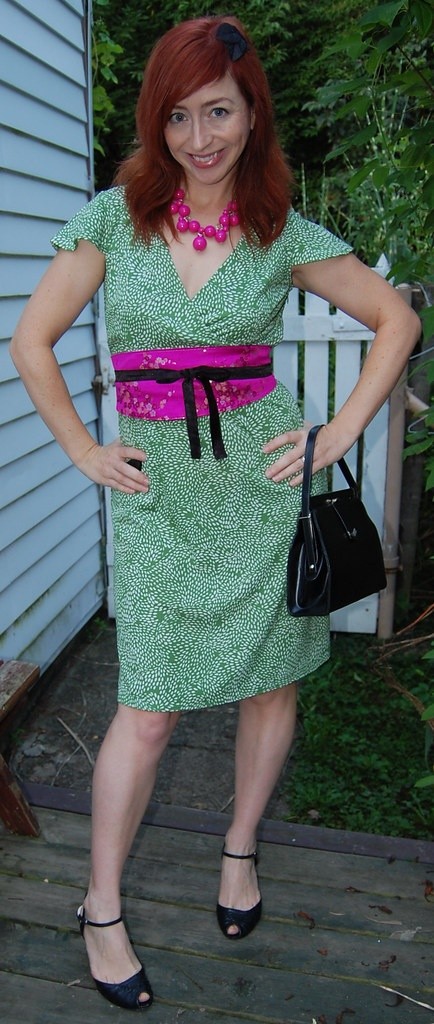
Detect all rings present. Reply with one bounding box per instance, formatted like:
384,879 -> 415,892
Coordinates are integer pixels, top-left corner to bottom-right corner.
300,455 -> 305,463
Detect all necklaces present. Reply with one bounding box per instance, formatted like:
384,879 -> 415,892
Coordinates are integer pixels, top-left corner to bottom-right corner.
169,188 -> 241,252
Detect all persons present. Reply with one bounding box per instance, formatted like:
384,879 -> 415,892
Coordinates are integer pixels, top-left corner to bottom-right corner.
10,18 -> 422,1009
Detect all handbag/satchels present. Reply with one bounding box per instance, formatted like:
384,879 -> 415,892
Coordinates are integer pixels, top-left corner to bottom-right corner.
287,424 -> 387,619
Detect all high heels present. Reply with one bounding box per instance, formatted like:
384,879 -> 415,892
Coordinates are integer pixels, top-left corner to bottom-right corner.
216,843 -> 261,940
76,893 -> 153,1010
